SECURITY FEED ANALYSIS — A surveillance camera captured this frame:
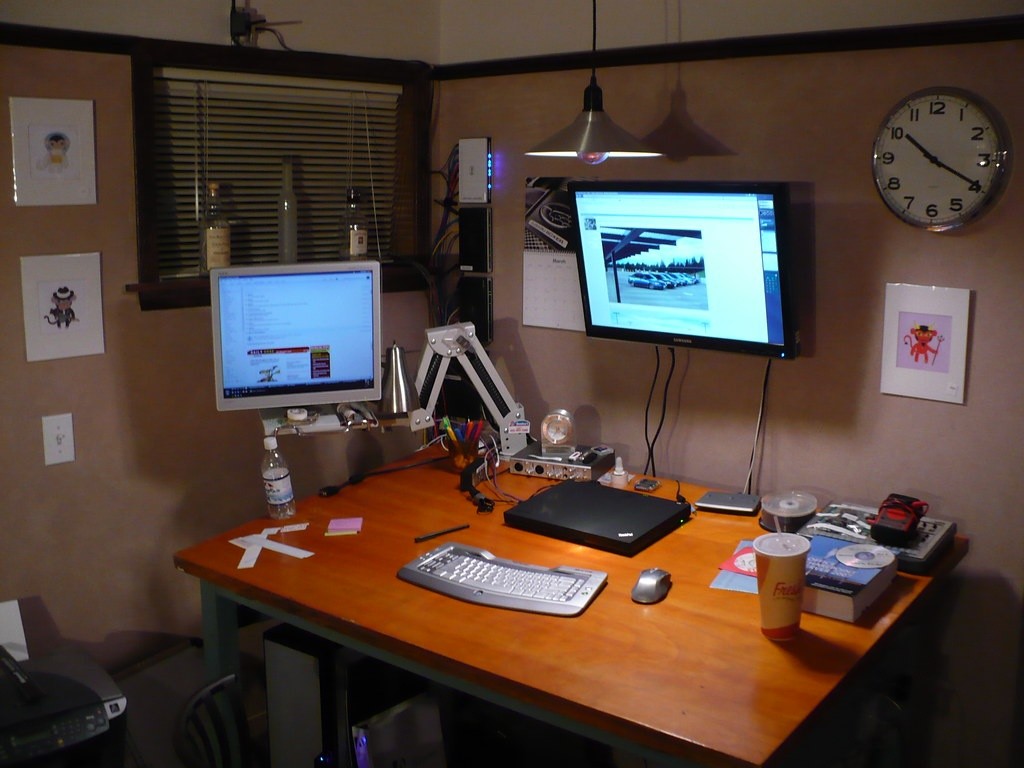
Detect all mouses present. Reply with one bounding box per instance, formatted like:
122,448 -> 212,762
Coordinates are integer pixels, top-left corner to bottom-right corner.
632,567 -> 671,603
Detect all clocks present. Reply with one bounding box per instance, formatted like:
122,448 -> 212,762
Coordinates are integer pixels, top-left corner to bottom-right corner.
872,86 -> 1015,233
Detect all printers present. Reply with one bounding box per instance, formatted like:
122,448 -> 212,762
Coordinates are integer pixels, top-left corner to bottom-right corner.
1,599 -> 132,768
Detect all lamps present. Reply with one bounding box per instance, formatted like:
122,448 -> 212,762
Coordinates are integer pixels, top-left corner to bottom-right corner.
382,321 -> 527,457
522,0 -> 666,164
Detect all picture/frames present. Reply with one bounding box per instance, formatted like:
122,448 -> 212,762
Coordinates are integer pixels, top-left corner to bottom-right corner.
8,96 -> 96,206
880,282 -> 970,405
19,252 -> 107,363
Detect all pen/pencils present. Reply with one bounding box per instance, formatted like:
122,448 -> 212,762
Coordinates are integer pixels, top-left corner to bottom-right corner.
443,416 -> 483,469
414,524 -> 470,542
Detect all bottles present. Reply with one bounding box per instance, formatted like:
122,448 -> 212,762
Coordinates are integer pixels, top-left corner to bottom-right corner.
611,455 -> 628,489
278,156 -> 299,266
260,436 -> 296,520
198,181 -> 230,274
339,189 -> 368,261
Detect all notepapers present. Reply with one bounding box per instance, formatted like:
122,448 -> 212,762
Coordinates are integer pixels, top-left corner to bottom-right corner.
328,518 -> 363,532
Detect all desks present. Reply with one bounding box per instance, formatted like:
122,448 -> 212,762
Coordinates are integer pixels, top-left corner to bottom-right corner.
173,453 -> 969,768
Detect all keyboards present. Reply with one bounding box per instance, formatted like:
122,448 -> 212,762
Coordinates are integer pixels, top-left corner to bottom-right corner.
396,542 -> 608,617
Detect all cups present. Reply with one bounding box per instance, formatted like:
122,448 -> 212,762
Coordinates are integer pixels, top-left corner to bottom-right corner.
752,532 -> 812,642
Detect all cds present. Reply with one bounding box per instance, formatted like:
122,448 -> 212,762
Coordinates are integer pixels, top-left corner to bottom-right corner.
835,544 -> 895,568
762,495 -> 816,517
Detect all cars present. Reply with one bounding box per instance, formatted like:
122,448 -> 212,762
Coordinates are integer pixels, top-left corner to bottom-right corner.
627,271 -> 701,290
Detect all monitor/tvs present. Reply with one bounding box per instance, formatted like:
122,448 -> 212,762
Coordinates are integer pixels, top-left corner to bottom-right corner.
209,260 -> 381,435
567,179 -> 799,360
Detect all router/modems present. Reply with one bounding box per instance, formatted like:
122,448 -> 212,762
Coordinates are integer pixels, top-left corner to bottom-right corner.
454,137 -> 495,344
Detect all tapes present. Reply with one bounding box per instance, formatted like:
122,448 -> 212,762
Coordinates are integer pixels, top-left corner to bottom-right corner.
286,408 -> 308,421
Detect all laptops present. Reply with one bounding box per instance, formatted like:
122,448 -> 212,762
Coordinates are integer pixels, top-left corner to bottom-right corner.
504,478 -> 691,557
